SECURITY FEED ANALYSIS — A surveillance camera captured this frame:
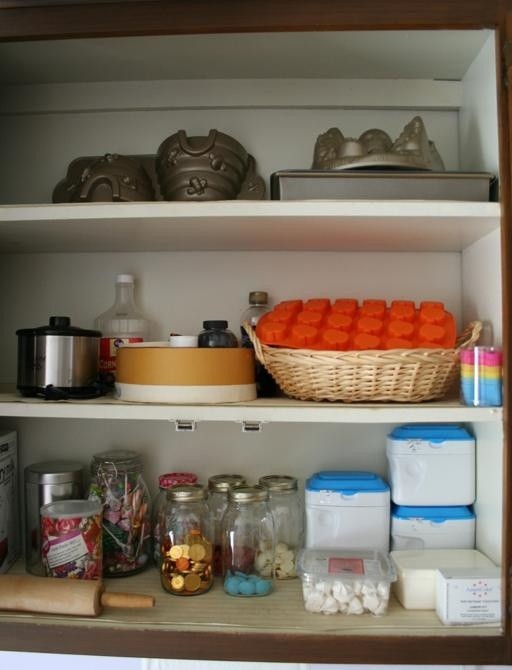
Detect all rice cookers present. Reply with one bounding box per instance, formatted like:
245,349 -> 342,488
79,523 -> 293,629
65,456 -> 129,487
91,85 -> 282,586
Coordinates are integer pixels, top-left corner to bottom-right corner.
14,314 -> 114,400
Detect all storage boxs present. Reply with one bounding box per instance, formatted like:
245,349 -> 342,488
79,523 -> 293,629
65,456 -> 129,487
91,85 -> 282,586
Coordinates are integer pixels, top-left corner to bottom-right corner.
387,506 -> 476,550
268,168 -> 500,204
386,422 -> 479,510
113,345 -> 258,405
0,427 -> 23,576
303,469 -> 390,558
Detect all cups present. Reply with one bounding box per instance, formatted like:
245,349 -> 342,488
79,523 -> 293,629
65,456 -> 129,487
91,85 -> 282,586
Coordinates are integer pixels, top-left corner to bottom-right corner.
169,335 -> 198,347
456,346 -> 503,406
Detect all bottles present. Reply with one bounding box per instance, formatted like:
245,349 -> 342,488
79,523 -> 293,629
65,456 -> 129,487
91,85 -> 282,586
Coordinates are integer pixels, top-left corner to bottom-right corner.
152,470 -> 302,597
93,272 -> 151,379
22,459 -> 85,577
198,320 -> 240,347
82,449 -> 154,579
240,290 -> 275,350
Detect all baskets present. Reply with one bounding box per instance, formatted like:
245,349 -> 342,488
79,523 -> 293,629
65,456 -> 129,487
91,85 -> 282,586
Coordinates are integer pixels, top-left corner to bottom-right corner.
242,318 -> 484,403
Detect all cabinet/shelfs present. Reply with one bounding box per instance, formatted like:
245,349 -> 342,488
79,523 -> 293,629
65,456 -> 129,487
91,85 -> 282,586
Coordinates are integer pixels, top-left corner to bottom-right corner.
0,0 -> 512,664
